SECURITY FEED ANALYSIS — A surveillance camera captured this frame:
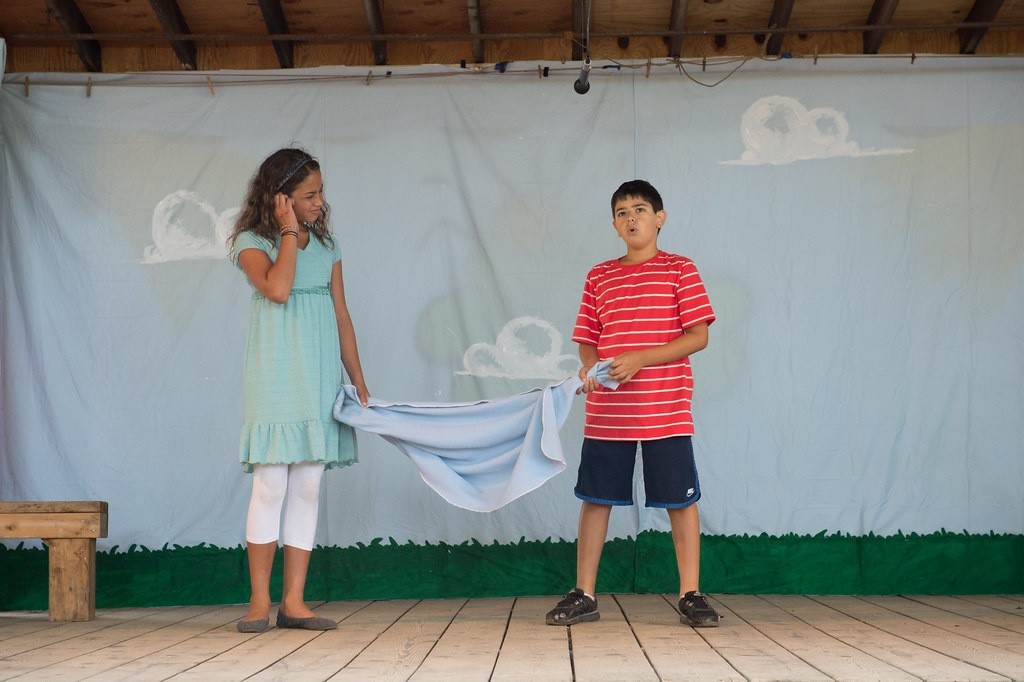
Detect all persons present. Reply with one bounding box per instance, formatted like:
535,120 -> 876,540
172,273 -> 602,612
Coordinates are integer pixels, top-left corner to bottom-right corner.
232,149 -> 370,633
545,179 -> 721,628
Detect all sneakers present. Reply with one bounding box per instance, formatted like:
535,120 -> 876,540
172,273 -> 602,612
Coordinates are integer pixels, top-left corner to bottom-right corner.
678,591 -> 721,627
545,587 -> 600,626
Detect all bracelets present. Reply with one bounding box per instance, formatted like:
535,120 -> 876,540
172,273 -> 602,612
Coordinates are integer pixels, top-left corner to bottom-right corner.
280,225 -> 299,239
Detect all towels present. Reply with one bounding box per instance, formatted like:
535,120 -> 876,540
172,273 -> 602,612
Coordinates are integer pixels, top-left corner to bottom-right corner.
331,358 -> 621,514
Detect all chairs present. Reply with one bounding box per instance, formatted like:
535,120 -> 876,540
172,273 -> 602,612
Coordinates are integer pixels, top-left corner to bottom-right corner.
0,496 -> 109,621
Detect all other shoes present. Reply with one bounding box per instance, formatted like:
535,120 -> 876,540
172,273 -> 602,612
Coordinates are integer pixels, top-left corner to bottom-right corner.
276,608 -> 338,630
236,617 -> 269,633
